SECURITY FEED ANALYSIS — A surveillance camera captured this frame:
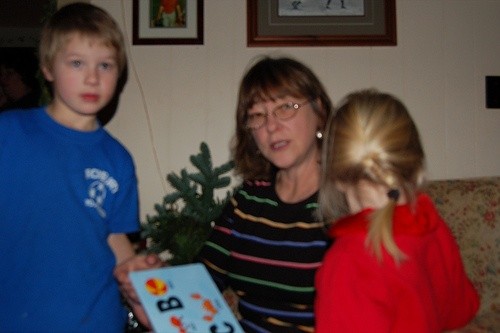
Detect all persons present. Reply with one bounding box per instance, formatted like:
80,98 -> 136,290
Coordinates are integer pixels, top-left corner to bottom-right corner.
1,1 -> 162,332
112,54 -> 346,332
157,0 -> 183,28
313,87 -> 481,333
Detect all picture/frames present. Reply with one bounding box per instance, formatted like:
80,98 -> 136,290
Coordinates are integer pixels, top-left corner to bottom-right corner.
246,0 -> 397,47
132,0 -> 204,45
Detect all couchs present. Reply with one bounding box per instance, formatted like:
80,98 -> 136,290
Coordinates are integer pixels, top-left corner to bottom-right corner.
422,178 -> 500,333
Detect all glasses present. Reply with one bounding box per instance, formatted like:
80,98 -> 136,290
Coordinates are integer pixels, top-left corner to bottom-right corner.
241,99 -> 313,129
